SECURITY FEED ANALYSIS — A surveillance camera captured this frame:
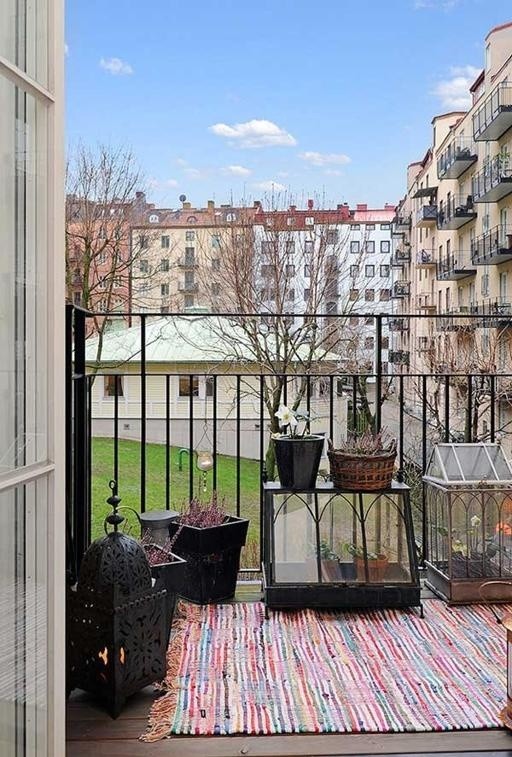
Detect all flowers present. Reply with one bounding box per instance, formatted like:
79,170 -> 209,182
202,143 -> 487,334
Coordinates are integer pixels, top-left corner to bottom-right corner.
137,522 -> 184,564
273,403 -> 315,438
176,488 -> 232,527
432,514 -> 493,557
326,424 -> 398,455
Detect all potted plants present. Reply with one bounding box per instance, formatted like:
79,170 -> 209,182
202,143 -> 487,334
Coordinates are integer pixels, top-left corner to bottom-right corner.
303,536 -> 388,583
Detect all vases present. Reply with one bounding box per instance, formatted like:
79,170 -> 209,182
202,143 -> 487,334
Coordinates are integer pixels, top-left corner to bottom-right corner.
270,433 -> 325,490
168,509 -> 250,605
144,543 -> 188,651
445,551 -> 490,577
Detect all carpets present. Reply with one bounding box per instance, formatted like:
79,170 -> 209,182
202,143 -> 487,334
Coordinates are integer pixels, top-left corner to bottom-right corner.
138,598 -> 511,743
